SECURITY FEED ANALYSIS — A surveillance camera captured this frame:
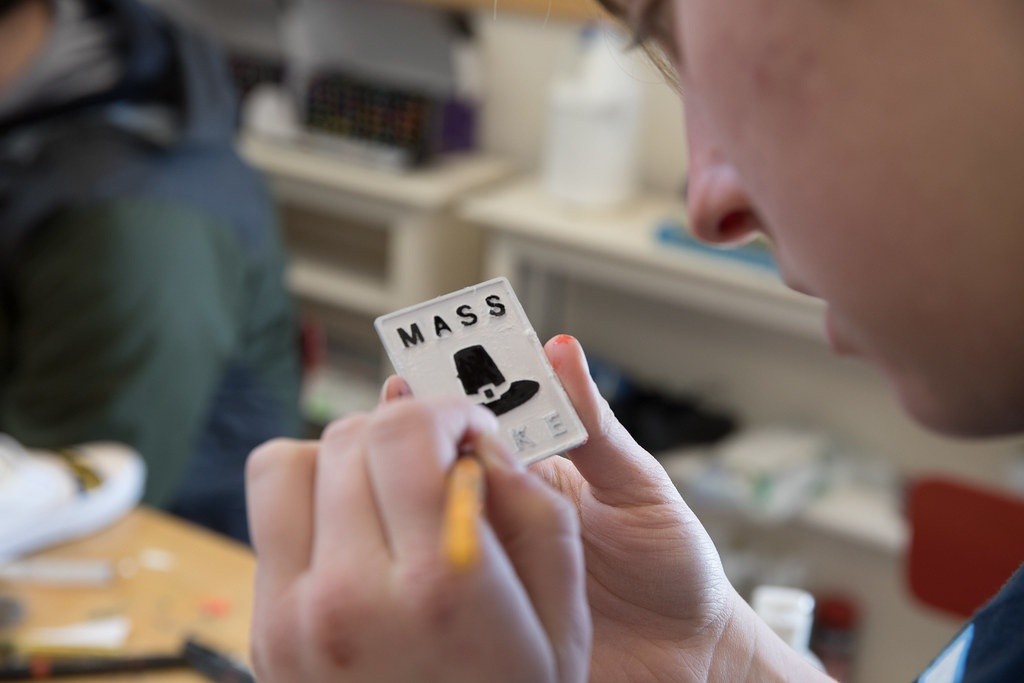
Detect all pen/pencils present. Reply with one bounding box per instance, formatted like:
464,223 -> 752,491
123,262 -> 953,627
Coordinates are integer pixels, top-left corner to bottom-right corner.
440,446 -> 490,568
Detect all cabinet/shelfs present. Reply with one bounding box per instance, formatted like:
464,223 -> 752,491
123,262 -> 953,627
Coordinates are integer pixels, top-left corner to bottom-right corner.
462,164 -> 1024,683
231,119 -> 515,442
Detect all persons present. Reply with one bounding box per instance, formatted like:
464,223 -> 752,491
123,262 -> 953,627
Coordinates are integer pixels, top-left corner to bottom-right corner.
1,0 -> 302,569
241,0 -> 1024,683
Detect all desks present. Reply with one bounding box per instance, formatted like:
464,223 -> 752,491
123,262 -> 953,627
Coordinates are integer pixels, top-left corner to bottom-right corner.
0,501 -> 258,683
